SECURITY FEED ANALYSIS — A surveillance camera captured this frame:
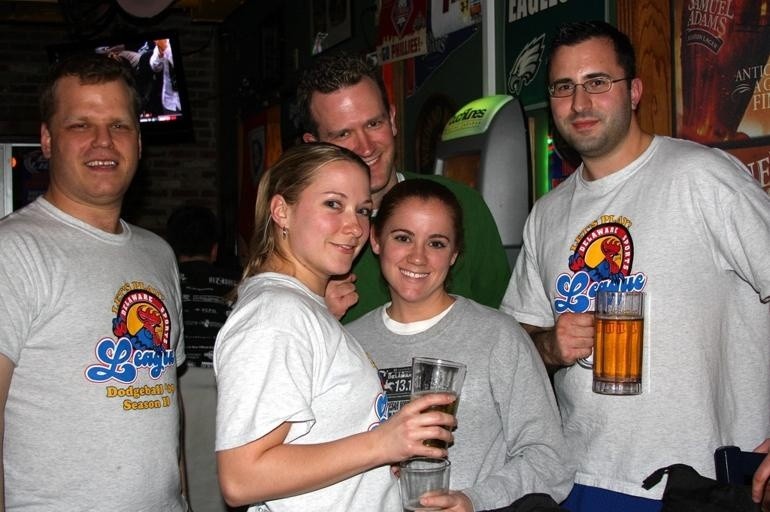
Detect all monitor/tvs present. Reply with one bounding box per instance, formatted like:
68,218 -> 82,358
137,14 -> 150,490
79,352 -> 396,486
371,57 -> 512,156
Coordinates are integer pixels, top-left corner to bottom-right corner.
92,35 -> 186,125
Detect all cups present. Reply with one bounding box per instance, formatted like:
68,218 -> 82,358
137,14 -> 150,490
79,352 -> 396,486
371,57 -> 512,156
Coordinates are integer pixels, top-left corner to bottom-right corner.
406,357 -> 466,462
400,458 -> 450,512
577,291 -> 644,397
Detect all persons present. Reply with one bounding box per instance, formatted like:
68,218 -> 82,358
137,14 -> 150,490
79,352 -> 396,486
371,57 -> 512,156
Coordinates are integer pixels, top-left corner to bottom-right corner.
341,177 -> 579,512
493,15 -> 769,512
138,38 -> 181,117
1,54 -> 193,512
295,46 -> 514,328
165,200 -> 236,512
208,138 -> 459,512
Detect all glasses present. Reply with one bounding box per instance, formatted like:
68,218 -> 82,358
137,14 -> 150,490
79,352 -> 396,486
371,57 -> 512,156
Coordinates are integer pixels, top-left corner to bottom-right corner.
544,77 -> 631,98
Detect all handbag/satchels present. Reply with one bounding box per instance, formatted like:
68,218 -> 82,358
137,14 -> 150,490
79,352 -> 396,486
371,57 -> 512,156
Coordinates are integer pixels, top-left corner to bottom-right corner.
641,463 -> 754,512
